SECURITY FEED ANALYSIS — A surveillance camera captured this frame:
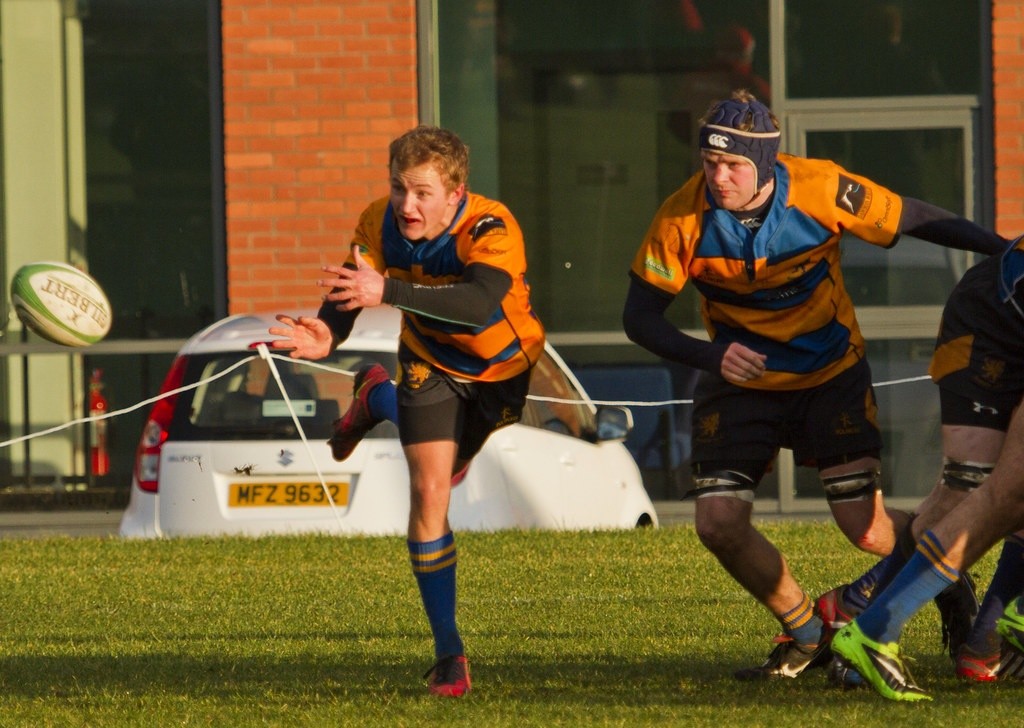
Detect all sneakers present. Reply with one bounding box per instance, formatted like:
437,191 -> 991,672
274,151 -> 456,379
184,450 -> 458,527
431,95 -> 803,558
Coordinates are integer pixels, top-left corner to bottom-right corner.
733,613 -> 833,683
956,630 -> 1024,686
327,362 -> 391,462
997,595 -> 1024,652
934,571 -> 982,659
423,653 -> 473,698
812,583 -> 861,643
829,616 -> 935,703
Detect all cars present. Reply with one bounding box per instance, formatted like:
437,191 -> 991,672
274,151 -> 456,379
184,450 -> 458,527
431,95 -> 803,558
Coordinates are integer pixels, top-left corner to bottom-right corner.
120,301 -> 660,540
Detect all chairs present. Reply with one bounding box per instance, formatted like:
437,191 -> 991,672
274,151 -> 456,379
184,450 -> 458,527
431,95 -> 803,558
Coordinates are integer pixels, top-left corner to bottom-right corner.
252,372 -> 338,438
574,363 -> 689,499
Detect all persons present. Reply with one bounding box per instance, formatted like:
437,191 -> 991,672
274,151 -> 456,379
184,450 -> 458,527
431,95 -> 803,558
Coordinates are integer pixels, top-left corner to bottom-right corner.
267,122 -> 546,698
620,87 -> 1024,701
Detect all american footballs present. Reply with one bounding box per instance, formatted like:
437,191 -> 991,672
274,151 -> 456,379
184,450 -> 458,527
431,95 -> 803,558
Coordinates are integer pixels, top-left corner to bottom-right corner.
8,259 -> 113,347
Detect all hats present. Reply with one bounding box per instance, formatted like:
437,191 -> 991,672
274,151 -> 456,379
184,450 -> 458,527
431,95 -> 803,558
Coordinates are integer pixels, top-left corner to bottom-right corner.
699,98 -> 782,196
